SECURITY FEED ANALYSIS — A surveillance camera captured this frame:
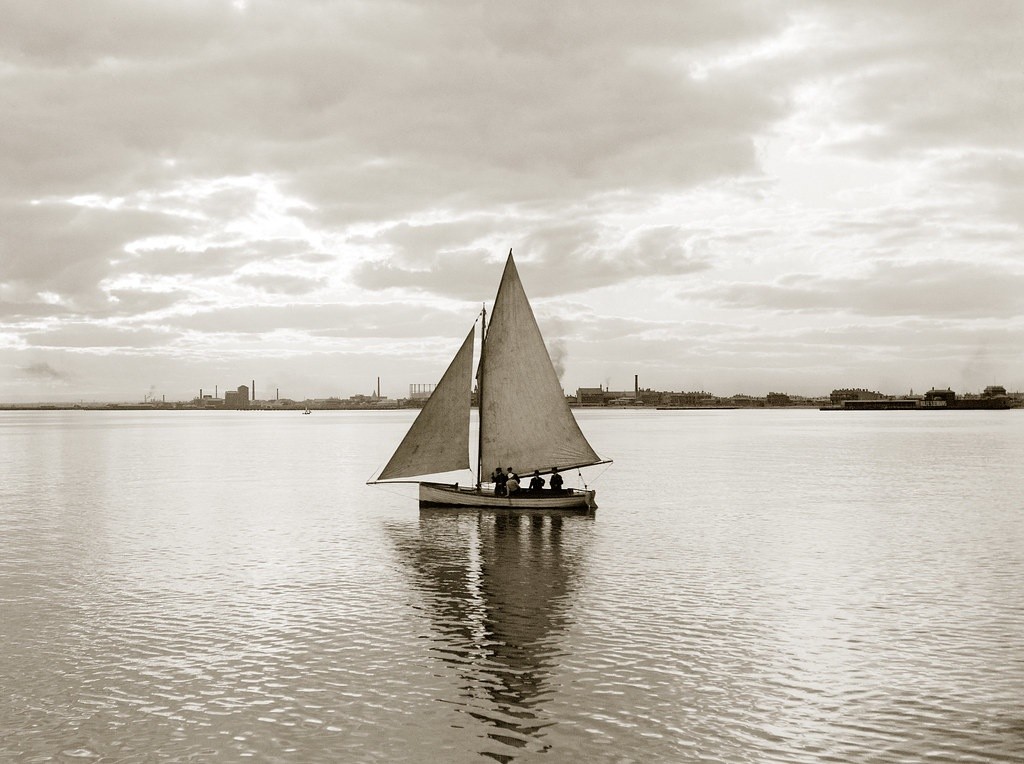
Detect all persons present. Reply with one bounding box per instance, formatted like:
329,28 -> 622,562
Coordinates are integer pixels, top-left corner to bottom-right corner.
529,470 -> 545,489
550,467 -> 563,496
491,467 -> 520,498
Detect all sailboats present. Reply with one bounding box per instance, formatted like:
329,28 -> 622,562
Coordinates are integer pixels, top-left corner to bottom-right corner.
366,247 -> 609,512
